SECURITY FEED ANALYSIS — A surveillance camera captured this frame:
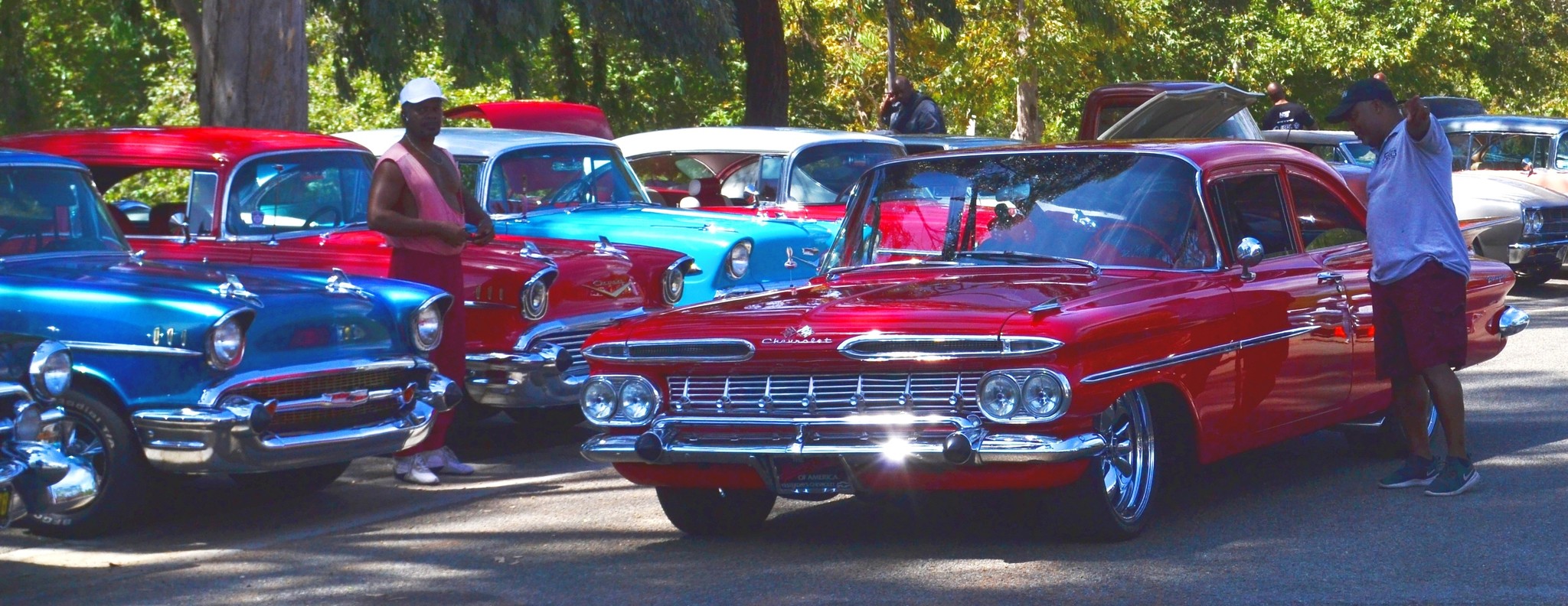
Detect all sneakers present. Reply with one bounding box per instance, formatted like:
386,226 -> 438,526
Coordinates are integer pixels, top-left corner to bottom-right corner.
393,453 -> 440,485
1377,455 -> 1440,491
1424,453 -> 1481,497
422,446 -> 475,475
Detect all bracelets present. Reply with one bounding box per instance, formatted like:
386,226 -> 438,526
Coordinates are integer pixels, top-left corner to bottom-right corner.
879,105 -> 888,112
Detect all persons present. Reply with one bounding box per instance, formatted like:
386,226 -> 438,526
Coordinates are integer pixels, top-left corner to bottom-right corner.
1263,81 -> 1317,149
367,77 -> 496,485
1327,79 -> 1482,496
878,75 -> 947,134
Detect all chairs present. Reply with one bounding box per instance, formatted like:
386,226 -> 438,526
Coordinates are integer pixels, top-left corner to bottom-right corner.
148,202 -> 212,235
680,177 -> 734,208
74,203 -> 139,234
611,180 -> 665,206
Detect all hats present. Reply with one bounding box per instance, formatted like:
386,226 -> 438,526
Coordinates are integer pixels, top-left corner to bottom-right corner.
400,78 -> 452,109
1326,78 -> 1394,125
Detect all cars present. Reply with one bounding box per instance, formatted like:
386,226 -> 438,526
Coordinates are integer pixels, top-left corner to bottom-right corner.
1,124 -> 697,463
443,79 -> 1568,294
0,147 -> 466,540
577,139 -> 1530,544
236,124 -> 881,309
